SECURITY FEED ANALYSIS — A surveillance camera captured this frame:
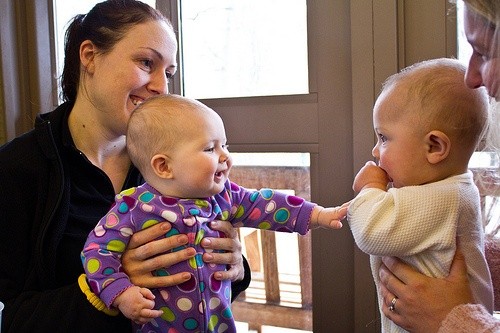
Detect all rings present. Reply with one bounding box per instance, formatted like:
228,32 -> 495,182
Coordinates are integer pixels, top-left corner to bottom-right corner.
388,296 -> 399,312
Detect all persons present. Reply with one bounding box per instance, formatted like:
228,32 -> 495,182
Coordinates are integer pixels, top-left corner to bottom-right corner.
0,0 -> 251,333
81,94 -> 352,333
378,0 -> 500,333
346,58 -> 493,333
